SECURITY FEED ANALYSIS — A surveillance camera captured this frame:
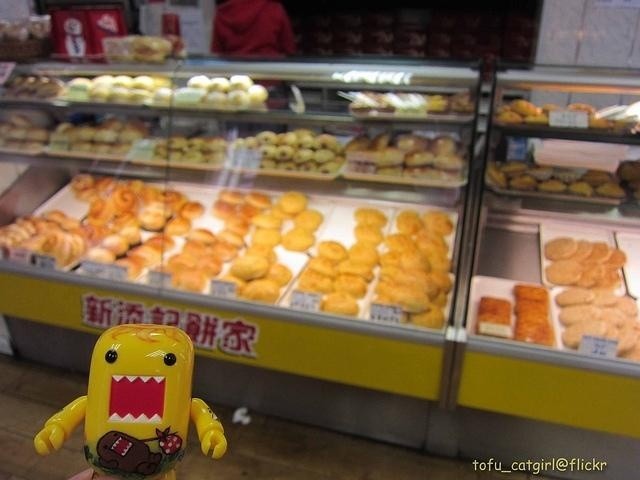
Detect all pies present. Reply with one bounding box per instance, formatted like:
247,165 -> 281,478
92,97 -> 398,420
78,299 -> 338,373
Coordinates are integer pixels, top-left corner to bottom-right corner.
477,236 -> 640,363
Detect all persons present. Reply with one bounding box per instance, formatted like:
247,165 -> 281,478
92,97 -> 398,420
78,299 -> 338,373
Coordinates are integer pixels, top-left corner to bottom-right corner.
210,0 -> 297,61
34,325 -> 228,480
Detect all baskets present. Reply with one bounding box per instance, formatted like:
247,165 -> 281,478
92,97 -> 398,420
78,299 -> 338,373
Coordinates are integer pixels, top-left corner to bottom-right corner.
0,38 -> 51,66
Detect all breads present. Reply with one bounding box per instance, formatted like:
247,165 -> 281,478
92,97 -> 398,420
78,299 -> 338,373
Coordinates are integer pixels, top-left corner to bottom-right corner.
1,15 -> 474,331
490,98 -> 640,207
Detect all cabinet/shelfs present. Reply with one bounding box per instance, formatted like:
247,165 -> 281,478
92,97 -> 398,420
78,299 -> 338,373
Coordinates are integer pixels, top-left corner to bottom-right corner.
0,56 -> 640,480
